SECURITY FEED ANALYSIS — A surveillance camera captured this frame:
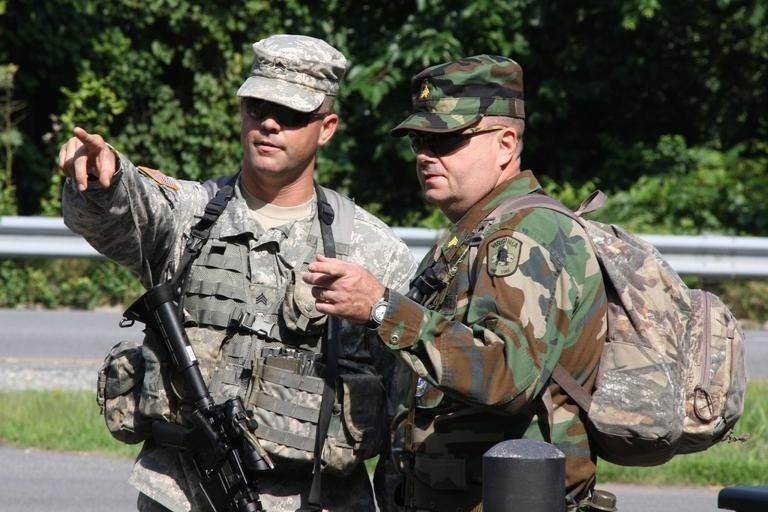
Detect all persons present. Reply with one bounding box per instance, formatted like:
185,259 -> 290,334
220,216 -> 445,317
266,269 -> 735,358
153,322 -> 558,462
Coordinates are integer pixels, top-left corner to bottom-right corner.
59,33 -> 421,512
302,54 -> 609,512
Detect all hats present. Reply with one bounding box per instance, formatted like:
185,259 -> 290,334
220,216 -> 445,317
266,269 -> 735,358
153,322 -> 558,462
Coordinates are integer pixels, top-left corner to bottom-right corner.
386,54 -> 524,136
237,34 -> 352,113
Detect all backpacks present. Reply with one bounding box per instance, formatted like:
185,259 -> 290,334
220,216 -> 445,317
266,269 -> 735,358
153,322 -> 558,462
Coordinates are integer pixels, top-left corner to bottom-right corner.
468,190 -> 745,466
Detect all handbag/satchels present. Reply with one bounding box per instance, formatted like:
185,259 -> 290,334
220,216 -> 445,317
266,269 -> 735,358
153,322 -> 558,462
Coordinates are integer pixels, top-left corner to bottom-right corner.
96,341 -> 146,444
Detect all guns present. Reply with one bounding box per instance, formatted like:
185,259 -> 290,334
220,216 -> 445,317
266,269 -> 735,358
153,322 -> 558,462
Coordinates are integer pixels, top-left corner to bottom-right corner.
119,284 -> 269,512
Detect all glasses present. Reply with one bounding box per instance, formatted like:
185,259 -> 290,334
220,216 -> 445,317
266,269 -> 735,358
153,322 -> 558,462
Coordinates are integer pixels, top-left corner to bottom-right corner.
243,98 -> 325,127
408,125 -> 519,154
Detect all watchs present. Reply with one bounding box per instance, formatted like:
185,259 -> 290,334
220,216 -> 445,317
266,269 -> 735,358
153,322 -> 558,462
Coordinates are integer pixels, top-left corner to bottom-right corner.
360,288 -> 389,331
